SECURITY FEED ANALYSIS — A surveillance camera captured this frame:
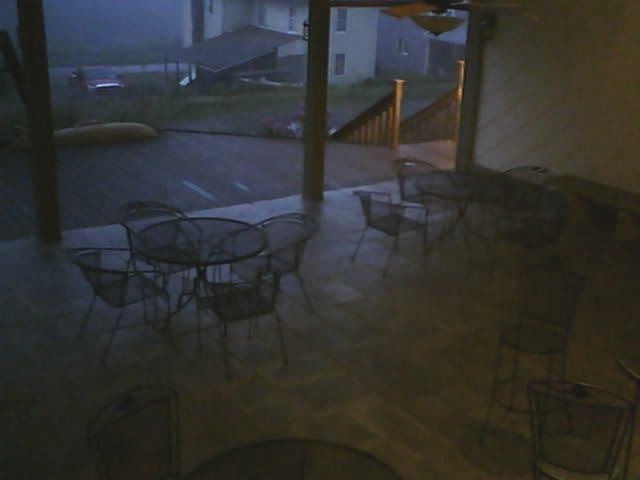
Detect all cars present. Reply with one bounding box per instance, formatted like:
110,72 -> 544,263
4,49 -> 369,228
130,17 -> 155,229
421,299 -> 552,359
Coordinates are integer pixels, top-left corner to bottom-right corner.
72,65 -> 125,98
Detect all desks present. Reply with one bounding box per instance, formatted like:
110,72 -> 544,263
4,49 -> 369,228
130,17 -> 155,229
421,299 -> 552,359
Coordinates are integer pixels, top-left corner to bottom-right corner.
184,438 -> 406,480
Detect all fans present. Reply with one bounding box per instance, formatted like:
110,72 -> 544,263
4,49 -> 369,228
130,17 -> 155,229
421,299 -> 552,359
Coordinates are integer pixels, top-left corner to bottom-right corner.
328,0 -> 534,20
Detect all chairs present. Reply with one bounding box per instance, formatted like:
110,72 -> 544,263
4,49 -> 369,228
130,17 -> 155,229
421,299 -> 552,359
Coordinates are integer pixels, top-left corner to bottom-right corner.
394,156 -> 459,242
525,378 -> 635,479
229,212 -> 319,311
192,263 -> 289,370
119,201 -> 204,289
415,158 -> 639,279
352,188 -> 429,274
83,384 -> 179,480
481,255 -> 575,452
68,247 -> 176,362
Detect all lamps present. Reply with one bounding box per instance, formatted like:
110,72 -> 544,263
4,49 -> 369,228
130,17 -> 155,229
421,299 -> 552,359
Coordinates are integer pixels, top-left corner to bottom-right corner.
407,10 -> 469,36
